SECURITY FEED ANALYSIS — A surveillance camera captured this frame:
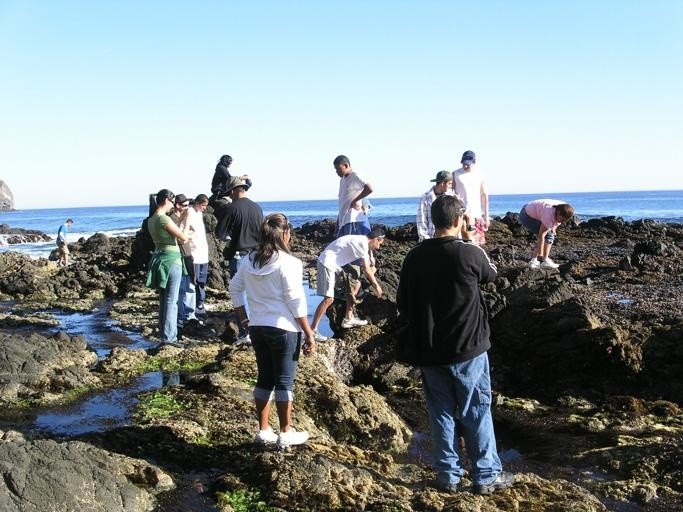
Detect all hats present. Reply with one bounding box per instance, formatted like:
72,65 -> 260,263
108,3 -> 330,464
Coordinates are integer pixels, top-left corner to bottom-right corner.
430,170 -> 452,182
461,150 -> 477,165
175,194 -> 192,203
223,176 -> 249,194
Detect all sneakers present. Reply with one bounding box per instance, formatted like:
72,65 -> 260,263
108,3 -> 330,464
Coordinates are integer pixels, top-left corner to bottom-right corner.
278,429 -> 309,446
255,428 -> 278,443
236,333 -> 251,345
528,259 -> 559,270
304,330 -> 328,342
477,472 -> 515,495
341,316 -> 368,328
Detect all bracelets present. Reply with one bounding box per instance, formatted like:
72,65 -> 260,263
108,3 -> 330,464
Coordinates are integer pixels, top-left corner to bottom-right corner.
241,319 -> 248,324
462,240 -> 472,243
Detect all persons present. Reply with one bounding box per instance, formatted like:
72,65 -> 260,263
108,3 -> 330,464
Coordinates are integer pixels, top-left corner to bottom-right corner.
518,198 -> 573,270
334,155 -> 373,299
416,171 -> 454,243
56,219 -> 74,266
212,155 -> 250,197
148,189 -> 210,347
228,213 -> 316,446
215,174 -> 263,346
312,228 -> 386,342
451,151 -> 490,244
397,195 -> 513,496
